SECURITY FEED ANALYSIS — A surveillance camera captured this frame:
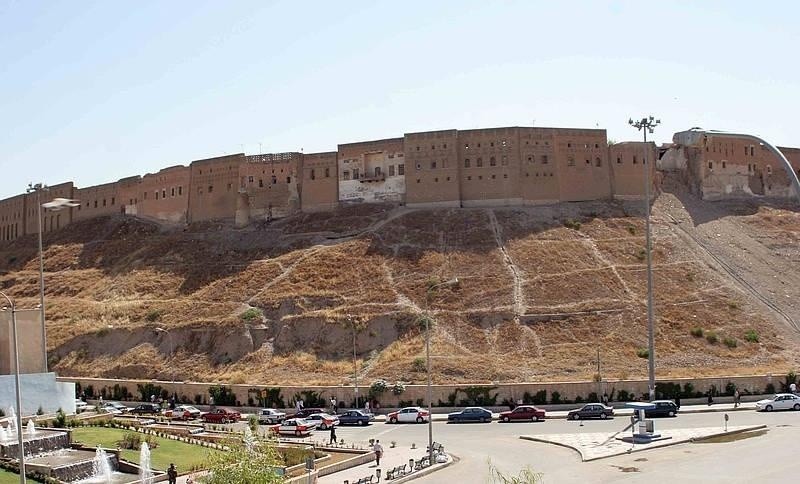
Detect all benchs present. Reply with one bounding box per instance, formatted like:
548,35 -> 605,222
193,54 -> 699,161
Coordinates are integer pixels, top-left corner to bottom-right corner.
386,464 -> 406,480
414,455 -> 431,470
426,442 -> 441,454
352,475 -> 373,484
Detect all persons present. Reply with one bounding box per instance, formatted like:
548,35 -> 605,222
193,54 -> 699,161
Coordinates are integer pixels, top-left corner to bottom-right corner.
104,384 -> 114,399
208,394 -> 214,410
135,389 -> 143,401
508,397 -> 515,410
373,439 -> 384,465
330,397 -> 338,414
675,392 -> 681,410
295,399 -> 305,414
150,393 -> 164,411
603,394 -> 609,406
169,394 -> 176,410
330,424 -> 337,444
167,462 -> 178,484
789,381 -> 797,394
272,399 -> 276,409
708,390 -> 713,406
364,399 -> 370,414
734,388 -> 740,407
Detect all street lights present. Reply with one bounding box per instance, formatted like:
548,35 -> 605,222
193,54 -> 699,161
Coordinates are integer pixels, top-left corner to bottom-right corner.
425,278 -> 459,466
155,327 -> 175,404
347,313 -> 359,408
672,127 -> 800,196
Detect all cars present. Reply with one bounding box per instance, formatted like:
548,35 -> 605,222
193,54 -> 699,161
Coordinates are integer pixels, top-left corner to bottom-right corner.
755,393 -> 800,412
386,406 -> 429,424
634,400 -> 678,418
200,407 -> 241,424
568,402 -> 615,420
499,406 -> 545,423
76,399 -> 161,417
162,405 -> 201,421
247,407 -> 375,438
448,406 -> 492,424
